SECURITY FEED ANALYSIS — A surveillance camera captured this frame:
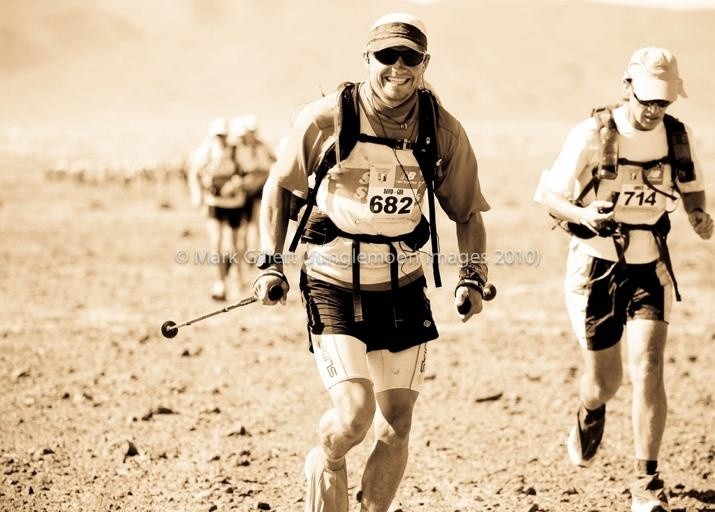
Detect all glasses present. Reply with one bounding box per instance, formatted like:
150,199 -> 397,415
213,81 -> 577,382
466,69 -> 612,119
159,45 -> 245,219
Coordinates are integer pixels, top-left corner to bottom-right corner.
633,92 -> 670,107
374,48 -> 424,66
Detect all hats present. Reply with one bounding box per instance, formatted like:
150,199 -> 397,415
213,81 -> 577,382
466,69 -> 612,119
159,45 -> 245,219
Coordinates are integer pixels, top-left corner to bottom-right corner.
624,47 -> 678,102
366,13 -> 427,53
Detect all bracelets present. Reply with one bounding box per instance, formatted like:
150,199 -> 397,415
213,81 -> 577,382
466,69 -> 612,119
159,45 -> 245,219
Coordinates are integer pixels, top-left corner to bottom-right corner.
458,256 -> 489,286
691,207 -> 704,213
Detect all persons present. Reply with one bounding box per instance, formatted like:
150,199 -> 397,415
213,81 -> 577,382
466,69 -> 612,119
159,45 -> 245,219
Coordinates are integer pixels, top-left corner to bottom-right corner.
252,12 -> 496,512
544,47 -> 715,512
231,116 -> 278,270
187,118 -> 251,301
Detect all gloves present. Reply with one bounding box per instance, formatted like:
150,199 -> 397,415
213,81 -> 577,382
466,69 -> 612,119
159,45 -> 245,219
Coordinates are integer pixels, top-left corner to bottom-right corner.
456,263 -> 488,322
252,267 -> 289,306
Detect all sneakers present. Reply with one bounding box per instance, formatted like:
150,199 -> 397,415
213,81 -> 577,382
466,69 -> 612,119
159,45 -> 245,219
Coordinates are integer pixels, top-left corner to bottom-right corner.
305,448 -> 348,512
631,472 -> 670,512
567,402 -> 605,468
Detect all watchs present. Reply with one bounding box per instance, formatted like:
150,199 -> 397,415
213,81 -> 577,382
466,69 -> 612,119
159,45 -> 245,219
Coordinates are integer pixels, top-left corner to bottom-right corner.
255,253 -> 283,270
